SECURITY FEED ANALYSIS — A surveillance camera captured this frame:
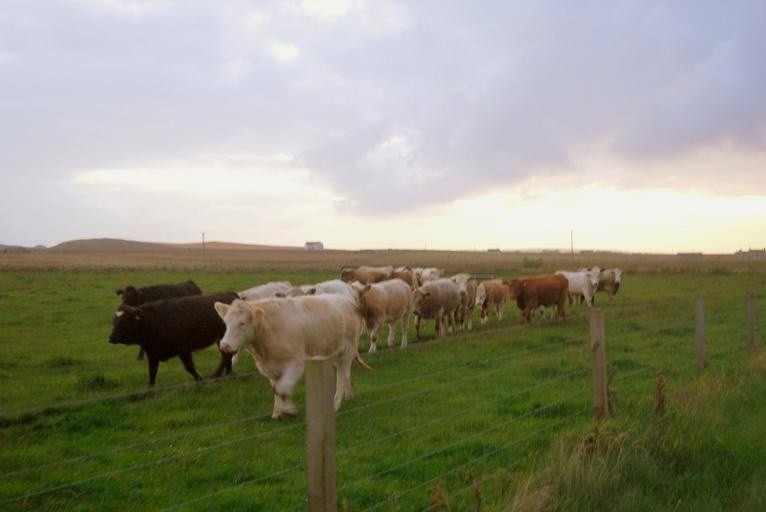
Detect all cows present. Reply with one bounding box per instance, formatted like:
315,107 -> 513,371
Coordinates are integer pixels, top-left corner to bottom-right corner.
238,279 -> 296,302
597,267 -> 625,305
116,280 -> 203,362
296,280 -> 361,299
106,290 -> 243,388
554,266 -> 606,309
411,278 -> 463,340
474,280 -> 508,325
508,274 -> 574,325
339,264 -> 443,285
349,278 -> 412,354
214,293 -> 374,421
